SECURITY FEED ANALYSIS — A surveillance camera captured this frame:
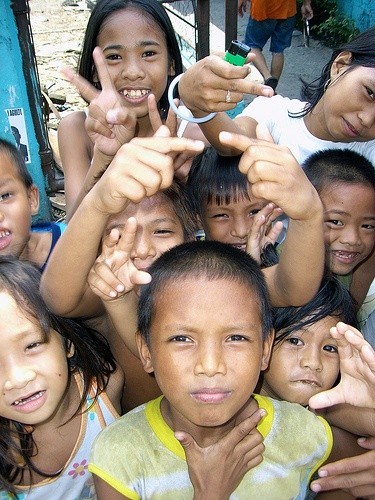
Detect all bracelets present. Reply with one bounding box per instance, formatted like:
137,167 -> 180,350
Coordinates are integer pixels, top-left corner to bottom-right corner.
167,69 -> 216,123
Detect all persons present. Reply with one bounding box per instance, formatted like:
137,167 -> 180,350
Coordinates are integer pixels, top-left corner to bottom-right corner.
88,240 -> 375,500
0,0 -> 375,500
0,253 -> 125,500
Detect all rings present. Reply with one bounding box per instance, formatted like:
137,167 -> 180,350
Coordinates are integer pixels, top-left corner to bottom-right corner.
226,89 -> 231,105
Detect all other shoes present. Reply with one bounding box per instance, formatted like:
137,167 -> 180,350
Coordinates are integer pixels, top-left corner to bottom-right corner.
262,75 -> 279,97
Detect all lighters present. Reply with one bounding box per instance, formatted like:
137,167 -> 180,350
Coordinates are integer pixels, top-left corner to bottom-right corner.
223,39 -> 251,66
